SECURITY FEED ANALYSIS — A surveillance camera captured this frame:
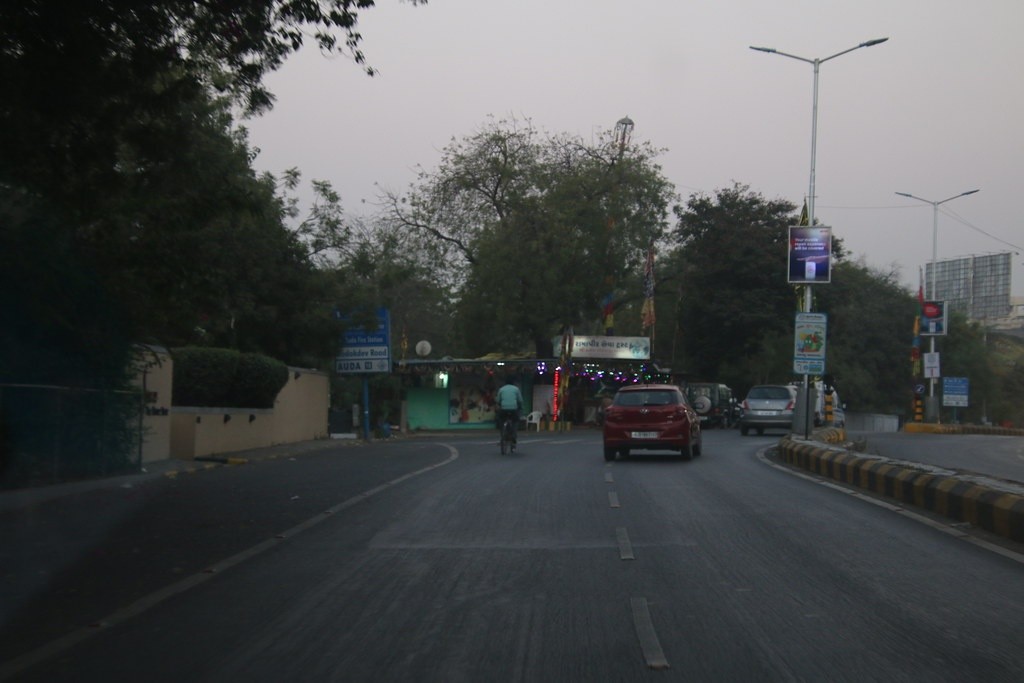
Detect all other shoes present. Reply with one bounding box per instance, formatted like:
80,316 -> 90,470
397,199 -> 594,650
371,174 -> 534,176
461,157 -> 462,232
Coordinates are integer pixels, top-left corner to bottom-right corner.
512,437 -> 516,444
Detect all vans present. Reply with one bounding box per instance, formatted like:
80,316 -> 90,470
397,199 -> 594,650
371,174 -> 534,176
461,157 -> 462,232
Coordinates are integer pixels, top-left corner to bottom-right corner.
790,381 -> 847,429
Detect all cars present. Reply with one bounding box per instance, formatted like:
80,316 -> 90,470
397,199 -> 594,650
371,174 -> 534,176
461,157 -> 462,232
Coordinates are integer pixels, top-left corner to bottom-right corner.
739,385 -> 797,435
601,383 -> 705,459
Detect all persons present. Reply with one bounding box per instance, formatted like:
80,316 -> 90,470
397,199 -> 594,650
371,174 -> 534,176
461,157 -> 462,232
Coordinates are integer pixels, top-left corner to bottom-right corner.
601,392 -> 612,410
497,377 -> 523,449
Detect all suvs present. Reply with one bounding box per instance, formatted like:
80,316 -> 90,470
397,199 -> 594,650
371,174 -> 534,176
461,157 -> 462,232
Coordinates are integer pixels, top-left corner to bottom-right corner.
681,383 -> 741,429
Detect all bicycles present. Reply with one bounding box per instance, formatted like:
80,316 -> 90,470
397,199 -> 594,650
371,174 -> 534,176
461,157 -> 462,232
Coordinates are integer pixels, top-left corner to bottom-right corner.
498,408 -> 522,455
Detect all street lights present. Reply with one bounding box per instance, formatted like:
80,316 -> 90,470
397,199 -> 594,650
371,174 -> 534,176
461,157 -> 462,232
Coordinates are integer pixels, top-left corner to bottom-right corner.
750,37 -> 889,439
896,189 -> 980,396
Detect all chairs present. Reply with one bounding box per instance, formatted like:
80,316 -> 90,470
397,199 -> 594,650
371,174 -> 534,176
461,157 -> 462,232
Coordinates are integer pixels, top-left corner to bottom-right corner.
526,411 -> 543,432
